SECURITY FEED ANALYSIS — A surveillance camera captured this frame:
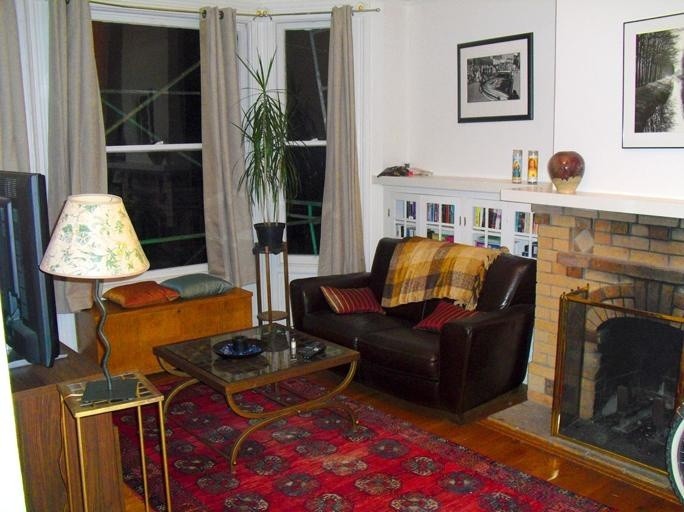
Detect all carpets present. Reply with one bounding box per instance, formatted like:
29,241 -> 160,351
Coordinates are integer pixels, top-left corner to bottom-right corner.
109,361 -> 617,512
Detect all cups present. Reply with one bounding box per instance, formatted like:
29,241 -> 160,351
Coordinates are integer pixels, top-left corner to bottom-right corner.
235,335 -> 248,351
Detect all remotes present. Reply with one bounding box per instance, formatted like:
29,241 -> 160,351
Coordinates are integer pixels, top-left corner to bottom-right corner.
297,341 -> 327,360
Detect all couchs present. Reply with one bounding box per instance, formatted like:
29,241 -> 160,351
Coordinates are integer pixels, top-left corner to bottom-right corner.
289,238 -> 536,418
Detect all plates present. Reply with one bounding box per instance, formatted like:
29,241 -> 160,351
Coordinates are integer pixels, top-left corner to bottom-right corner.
214,341 -> 264,359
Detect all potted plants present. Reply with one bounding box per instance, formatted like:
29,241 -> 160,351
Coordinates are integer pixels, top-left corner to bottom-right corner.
232,45 -> 310,251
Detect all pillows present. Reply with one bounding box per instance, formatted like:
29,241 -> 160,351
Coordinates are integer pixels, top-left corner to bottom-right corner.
319,283 -> 384,315
413,296 -> 482,337
100,273 -> 230,309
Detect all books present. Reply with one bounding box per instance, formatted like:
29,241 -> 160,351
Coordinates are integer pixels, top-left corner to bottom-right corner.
394,198 -> 538,261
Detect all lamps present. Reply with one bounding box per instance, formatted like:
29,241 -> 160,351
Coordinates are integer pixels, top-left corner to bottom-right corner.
37,194 -> 150,407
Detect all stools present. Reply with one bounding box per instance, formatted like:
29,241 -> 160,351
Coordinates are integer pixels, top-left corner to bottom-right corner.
56,370 -> 171,512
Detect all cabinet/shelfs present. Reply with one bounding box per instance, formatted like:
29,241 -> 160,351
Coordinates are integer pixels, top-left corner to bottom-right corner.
74,287 -> 253,376
373,170 -> 537,262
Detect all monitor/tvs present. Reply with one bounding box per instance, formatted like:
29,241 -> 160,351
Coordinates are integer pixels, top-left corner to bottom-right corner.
0,171 -> 68,369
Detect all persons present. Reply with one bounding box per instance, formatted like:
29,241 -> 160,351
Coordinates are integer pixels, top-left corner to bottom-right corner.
527,158 -> 538,179
512,159 -> 521,180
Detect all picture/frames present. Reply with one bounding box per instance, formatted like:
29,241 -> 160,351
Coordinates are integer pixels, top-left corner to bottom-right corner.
456,33 -> 533,124
621,11 -> 684,148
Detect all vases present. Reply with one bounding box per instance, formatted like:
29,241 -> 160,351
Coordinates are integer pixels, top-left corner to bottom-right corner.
548,152 -> 584,194
